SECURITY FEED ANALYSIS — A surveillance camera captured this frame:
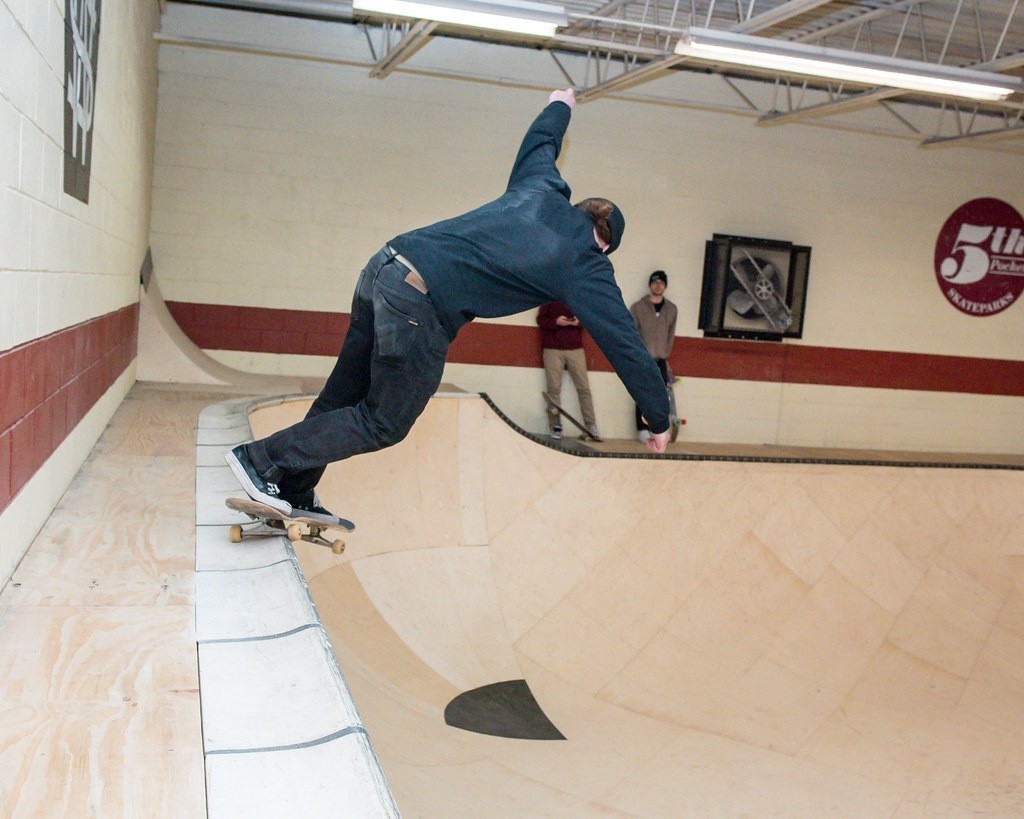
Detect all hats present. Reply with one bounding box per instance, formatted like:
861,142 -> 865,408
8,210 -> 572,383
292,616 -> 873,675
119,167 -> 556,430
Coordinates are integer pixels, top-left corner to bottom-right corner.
649,270 -> 669,290
596,196 -> 625,256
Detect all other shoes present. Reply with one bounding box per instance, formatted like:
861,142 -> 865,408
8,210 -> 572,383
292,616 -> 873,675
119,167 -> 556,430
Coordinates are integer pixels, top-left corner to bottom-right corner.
548,426 -> 564,438
587,432 -> 603,443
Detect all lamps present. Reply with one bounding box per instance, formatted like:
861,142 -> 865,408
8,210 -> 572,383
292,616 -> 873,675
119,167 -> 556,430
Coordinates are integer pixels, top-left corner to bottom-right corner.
672,1 -> 1024,103
352,0 -> 570,38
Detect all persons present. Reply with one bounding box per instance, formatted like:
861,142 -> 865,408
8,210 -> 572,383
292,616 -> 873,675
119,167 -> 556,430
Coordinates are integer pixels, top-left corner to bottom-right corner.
535,300 -> 605,443
224,86 -> 673,519
626,268 -> 679,443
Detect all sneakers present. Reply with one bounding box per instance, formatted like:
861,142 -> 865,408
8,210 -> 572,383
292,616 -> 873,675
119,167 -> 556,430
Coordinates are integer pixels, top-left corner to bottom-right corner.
636,430 -> 652,443
287,492 -> 339,526
228,444 -> 292,513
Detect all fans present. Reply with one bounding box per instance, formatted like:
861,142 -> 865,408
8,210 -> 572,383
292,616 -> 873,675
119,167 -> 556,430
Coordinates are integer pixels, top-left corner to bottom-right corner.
698,233 -> 814,342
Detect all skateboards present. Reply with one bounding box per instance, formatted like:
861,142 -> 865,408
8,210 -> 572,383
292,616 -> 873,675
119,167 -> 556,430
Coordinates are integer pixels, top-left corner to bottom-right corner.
224,498 -> 357,556
541,391 -> 604,443
662,363 -> 688,442
